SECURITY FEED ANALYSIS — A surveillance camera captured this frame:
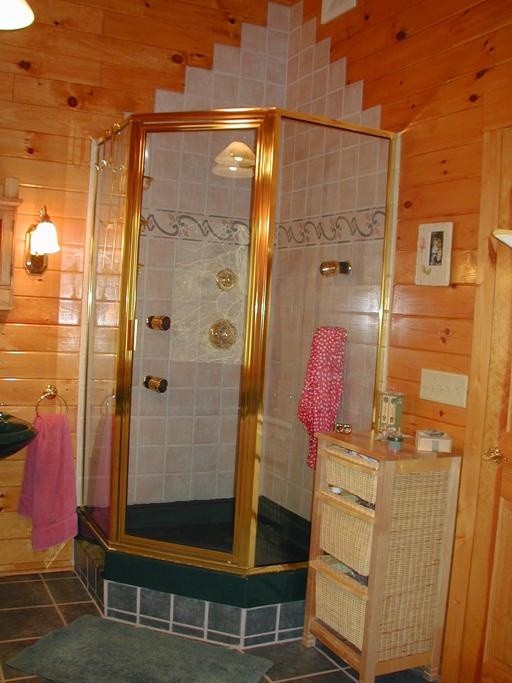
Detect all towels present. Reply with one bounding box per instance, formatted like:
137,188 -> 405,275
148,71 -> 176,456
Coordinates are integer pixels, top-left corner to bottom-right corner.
88,417 -> 112,539
17,416 -> 79,554
297,328 -> 346,470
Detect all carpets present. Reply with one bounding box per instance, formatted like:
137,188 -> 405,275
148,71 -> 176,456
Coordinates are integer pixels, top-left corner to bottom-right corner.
6,614 -> 276,682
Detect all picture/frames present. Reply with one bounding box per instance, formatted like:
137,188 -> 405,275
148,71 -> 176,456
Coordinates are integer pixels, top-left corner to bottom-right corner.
413,221 -> 454,287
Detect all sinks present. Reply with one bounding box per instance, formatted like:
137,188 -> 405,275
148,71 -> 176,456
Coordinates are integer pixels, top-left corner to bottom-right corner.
0,413 -> 37,459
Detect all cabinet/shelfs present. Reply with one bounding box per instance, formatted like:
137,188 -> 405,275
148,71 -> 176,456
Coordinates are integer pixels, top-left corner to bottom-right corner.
302,428 -> 464,683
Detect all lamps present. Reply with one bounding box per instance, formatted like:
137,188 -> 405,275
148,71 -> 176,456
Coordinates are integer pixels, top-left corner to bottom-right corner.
25,206 -> 62,275
211,142 -> 256,180
